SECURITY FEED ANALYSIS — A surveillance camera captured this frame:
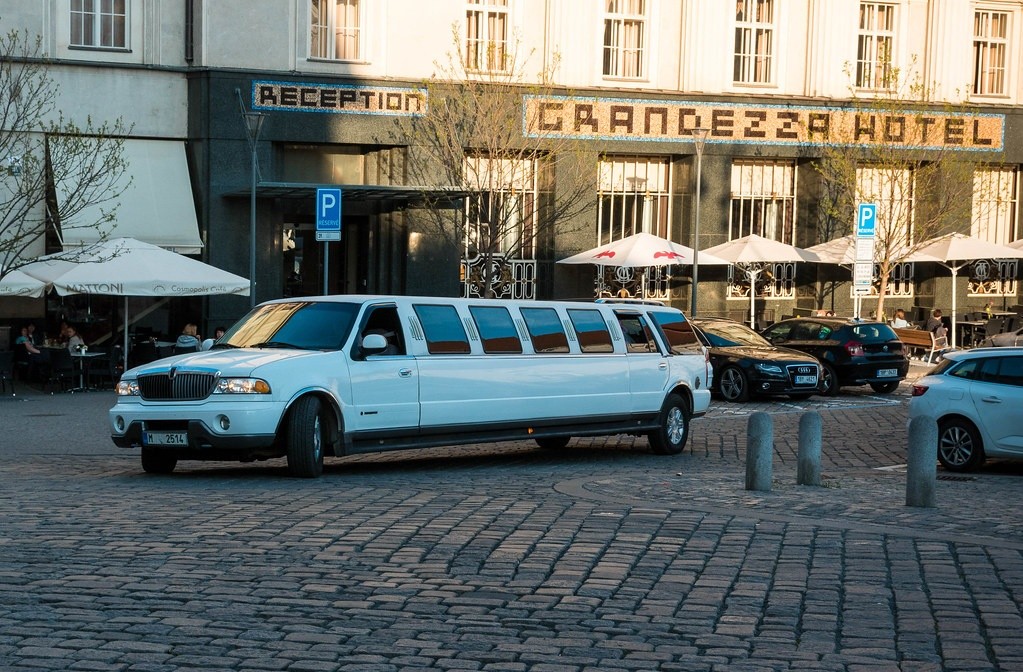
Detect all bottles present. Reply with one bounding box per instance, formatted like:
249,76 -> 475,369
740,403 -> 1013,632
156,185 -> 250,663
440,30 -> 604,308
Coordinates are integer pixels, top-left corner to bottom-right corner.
149,337 -> 158,345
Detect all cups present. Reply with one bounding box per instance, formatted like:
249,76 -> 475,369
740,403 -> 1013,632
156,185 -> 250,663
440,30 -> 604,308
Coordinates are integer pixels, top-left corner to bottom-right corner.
48,339 -> 52,345
54,340 -> 57,345
62,343 -> 66,348
81,349 -> 85,355
45,340 -> 48,345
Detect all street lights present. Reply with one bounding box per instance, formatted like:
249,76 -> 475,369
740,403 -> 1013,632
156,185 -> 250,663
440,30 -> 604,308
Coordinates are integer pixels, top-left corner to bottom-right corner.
241,108 -> 271,309
690,126 -> 712,317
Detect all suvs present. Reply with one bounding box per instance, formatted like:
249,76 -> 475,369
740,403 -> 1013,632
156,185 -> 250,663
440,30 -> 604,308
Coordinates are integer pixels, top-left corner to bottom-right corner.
760,316 -> 911,398
906,345 -> 1023,474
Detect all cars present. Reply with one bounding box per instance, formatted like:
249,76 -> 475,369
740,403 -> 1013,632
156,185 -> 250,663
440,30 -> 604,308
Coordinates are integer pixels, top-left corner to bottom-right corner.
107,293 -> 715,478
984,328 -> 1023,348
686,316 -> 825,402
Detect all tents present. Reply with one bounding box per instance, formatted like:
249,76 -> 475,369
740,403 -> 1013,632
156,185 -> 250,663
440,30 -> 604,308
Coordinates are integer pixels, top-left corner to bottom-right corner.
0,237 -> 256,373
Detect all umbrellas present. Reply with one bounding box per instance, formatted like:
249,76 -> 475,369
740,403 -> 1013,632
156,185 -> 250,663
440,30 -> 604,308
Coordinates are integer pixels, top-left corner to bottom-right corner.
557,231 -> 1023,358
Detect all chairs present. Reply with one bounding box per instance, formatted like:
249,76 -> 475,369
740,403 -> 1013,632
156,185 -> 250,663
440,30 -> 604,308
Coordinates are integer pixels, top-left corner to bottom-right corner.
788,326 -> 810,339
941,305 -> 1023,350
0,350 -> 16,397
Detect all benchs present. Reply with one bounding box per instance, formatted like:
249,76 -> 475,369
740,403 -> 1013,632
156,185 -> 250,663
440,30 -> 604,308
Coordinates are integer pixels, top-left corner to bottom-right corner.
891,328 -> 950,366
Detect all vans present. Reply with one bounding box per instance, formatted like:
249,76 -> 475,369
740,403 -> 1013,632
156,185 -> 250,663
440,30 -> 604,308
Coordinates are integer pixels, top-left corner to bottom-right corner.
593,298 -> 665,306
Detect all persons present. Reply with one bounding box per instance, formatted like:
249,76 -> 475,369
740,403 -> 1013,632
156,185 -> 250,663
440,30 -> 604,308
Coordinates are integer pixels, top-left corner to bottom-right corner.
891,309 -> 912,358
175,323 -> 227,352
983,303 -> 992,323
922,309 -> 947,363
18,320 -> 86,390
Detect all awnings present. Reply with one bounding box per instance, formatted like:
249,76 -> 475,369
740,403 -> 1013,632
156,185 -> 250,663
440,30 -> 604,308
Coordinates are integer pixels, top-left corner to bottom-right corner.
0,132 -> 204,273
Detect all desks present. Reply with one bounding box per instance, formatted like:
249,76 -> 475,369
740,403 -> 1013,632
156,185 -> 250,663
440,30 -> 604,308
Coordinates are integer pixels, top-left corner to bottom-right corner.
141,340 -> 176,360
977,309 -> 1018,319
68,351 -> 106,393
29,346 -> 73,396
956,320 -> 988,349
